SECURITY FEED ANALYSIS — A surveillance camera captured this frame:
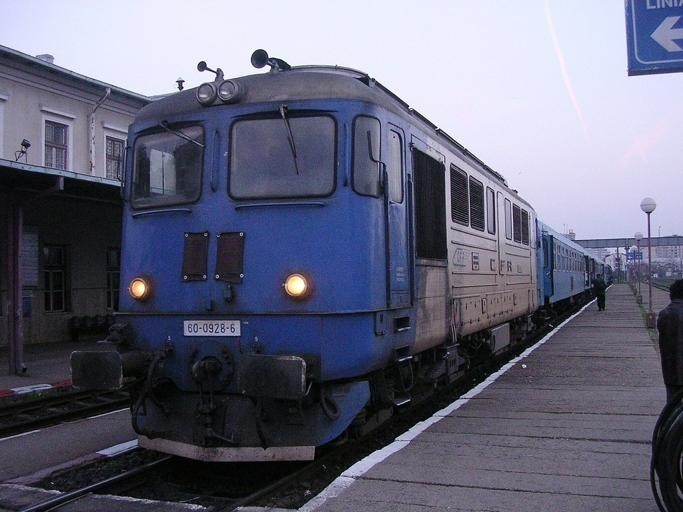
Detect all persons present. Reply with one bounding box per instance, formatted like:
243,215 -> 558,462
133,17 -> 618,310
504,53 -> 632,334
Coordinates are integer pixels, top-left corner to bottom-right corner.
656,278 -> 682,403
593,272 -> 606,311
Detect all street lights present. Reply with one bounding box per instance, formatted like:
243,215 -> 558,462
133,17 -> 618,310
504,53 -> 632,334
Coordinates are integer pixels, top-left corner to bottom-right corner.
638,196 -> 657,329
634,231 -> 644,303
624,245 -> 638,294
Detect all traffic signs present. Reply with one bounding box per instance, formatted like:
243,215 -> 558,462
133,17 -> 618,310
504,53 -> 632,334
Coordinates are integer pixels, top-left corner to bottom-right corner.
623,0 -> 683,76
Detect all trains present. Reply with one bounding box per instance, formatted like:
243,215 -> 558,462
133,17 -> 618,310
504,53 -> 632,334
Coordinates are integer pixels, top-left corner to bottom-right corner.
68,50 -> 616,498
